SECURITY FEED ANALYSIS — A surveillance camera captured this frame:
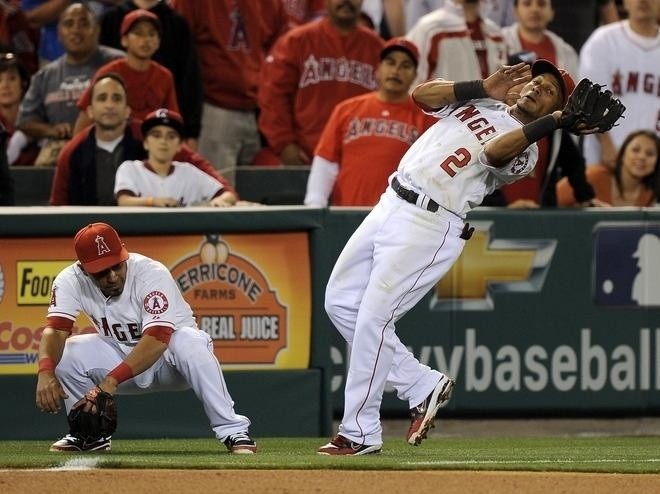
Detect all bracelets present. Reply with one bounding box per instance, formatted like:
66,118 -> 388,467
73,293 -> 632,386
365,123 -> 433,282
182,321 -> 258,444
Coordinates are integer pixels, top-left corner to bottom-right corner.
38,357 -> 58,372
105,362 -> 133,383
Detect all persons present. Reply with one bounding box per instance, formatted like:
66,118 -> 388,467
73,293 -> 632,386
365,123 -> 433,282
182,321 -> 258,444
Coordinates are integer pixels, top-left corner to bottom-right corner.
313,58 -> 626,459
33,220 -> 255,453
1,2 -> 659,208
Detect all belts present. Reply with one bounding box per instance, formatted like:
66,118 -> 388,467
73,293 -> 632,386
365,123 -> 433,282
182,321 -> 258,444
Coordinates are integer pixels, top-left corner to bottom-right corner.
392,176 -> 439,213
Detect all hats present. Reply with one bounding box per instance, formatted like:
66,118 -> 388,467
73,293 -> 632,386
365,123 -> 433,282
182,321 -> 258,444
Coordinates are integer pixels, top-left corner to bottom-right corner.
379,38 -> 418,66
532,59 -> 575,108
74,222 -> 129,274
141,108 -> 185,136
120,9 -> 158,34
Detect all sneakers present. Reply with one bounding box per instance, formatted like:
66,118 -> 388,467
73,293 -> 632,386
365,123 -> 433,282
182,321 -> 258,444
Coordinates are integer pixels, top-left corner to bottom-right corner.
317,434 -> 382,456
407,374 -> 455,446
224,432 -> 257,454
49,433 -> 112,452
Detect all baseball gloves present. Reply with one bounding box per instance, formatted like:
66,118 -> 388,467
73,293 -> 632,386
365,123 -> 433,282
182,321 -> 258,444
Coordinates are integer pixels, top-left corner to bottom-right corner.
554,79 -> 626,136
67,388 -> 120,441
